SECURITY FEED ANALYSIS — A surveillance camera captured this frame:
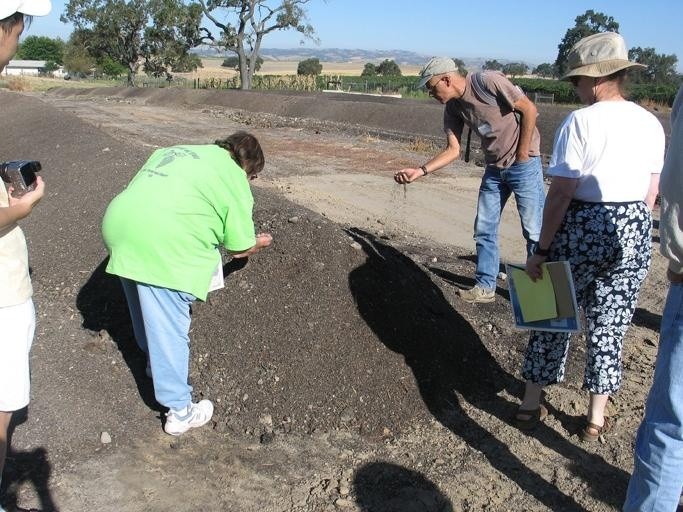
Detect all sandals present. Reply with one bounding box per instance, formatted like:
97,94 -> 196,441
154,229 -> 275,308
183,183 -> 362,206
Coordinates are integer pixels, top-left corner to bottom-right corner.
581,416 -> 612,442
514,406 -> 550,429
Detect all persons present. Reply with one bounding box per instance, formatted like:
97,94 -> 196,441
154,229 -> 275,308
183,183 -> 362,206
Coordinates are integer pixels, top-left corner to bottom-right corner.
0,1 -> 54,481
622,76 -> 683,512
101,131 -> 273,438
393,56 -> 546,305
514,32 -> 665,446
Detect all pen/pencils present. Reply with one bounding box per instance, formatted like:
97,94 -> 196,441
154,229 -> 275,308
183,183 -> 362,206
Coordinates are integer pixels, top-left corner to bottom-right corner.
507,263 -> 526,273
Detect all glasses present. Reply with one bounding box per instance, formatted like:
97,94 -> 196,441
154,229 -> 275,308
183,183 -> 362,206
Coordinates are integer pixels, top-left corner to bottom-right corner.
250,173 -> 258,180
425,79 -> 443,92
569,75 -> 581,87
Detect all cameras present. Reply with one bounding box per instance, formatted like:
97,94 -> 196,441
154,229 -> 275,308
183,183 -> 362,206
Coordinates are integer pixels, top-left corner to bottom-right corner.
1,159 -> 41,195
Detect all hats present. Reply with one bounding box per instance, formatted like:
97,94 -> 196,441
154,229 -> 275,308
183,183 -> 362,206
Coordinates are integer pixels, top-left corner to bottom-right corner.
412,57 -> 459,90
0,0 -> 52,20
558,31 -> 649,78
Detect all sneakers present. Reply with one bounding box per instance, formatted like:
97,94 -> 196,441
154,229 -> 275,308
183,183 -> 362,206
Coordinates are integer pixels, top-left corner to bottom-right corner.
164,399 -> 214,435
145,358 -> 152,378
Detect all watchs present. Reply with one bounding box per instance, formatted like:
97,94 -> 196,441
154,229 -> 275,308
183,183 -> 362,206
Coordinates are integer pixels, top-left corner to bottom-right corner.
419,166 -> 427,176
533,243 -> 547,257
